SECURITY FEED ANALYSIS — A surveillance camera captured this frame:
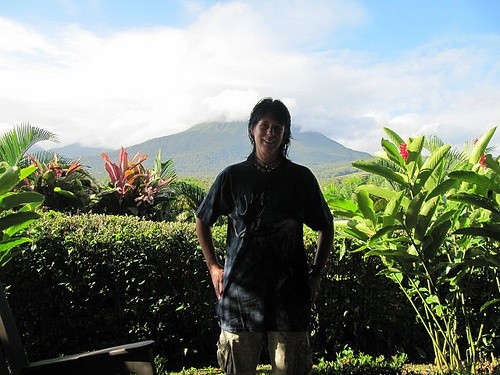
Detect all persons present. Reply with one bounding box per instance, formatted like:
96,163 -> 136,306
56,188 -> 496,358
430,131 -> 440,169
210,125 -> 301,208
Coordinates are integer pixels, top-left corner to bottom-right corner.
196,98 -> 336,375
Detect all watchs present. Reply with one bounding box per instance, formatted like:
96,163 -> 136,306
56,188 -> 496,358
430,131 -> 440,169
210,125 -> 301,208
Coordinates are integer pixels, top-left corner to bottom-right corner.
311,265 -> 323,273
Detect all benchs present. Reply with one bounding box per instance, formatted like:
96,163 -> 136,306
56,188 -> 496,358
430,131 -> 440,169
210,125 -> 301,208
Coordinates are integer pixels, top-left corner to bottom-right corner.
0,289 -> 158,374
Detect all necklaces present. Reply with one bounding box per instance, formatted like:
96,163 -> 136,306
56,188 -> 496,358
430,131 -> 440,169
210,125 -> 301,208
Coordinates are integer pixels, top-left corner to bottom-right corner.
252,156 -> 282,172
